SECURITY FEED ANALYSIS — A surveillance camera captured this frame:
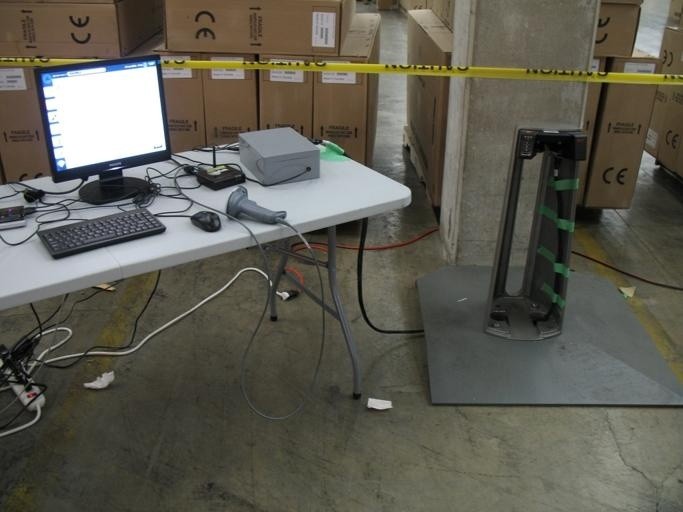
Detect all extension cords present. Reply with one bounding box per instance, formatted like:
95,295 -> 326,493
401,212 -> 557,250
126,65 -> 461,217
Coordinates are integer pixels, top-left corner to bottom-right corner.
0,354 -> 46,412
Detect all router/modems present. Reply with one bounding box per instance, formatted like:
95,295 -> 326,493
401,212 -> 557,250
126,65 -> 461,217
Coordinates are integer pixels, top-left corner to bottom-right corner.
196,145 -> 246,191
199,163 -> 244,192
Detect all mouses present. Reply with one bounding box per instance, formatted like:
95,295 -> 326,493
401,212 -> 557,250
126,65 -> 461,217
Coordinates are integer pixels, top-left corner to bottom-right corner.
191,210 -> 221,232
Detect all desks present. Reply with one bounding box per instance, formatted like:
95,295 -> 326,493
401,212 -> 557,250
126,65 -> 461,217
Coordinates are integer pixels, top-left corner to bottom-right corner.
0,141 -> 413,401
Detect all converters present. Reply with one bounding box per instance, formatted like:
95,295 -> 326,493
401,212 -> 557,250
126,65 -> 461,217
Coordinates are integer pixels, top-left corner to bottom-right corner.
0,206 -> 27,230
0,344 -> 9,359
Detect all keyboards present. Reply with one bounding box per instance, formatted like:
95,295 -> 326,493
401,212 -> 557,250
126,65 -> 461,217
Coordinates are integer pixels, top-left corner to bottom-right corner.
36,207 -> 167,260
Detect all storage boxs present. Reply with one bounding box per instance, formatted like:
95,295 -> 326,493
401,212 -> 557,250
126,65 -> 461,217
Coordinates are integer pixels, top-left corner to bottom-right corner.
584,56 -> 665,210
404,7 -> 453,205
312,10 -> 380,170
200,56 -> 257,147
256,57 -> 312,146
588,2 -> 644,58
1,1 -> 161,59
128,31 -> 206,153
642,2 -> 682,180
1,66 -> 50,186
575,57 -> 608,207
165,2 -> 356,56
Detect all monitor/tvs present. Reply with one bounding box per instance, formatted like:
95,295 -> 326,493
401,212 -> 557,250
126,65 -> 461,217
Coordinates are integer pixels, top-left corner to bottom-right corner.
33,54 -> 172,206
